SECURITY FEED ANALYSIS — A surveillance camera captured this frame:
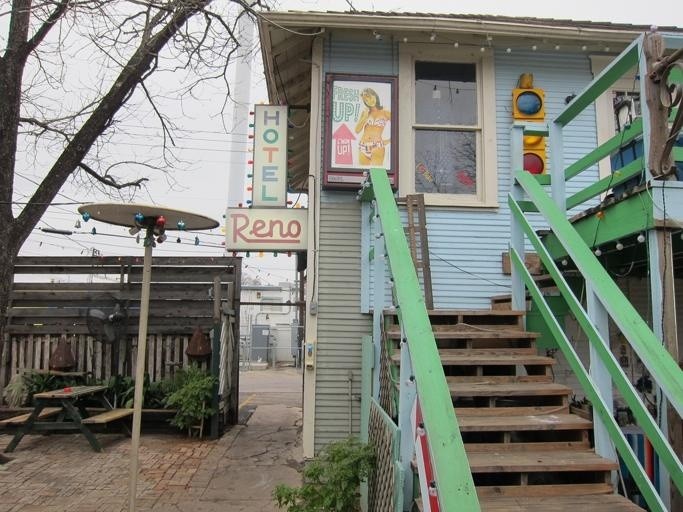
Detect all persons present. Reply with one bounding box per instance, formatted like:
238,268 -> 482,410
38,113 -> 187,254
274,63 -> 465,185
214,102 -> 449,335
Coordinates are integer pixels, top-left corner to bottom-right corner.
355,88 -> 391,166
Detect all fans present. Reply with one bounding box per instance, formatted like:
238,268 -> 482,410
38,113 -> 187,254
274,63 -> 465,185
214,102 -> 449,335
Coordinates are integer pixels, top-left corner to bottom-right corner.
87,294 -> 128,376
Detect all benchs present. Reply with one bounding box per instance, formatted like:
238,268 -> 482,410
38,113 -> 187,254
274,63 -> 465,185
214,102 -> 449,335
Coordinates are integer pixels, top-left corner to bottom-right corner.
80,409 -> 133,428
0,407 -> 63,427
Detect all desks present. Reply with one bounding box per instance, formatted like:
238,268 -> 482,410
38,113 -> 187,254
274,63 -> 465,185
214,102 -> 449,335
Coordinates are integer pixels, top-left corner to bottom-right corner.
3,386 -> 132,452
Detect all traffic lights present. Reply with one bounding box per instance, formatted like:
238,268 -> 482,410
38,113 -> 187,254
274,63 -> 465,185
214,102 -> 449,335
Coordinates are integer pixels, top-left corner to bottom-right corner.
511,88 -> 545,176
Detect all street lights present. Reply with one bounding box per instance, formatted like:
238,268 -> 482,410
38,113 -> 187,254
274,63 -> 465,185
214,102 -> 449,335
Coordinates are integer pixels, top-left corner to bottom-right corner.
73,201 -> 220,512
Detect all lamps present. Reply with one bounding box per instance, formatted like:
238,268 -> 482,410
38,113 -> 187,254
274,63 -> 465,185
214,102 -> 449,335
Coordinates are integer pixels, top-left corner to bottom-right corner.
75,213 -> 185,244
595,248 -> 602,256
372,31 -> 611,57
637,233 -> 645,243
562,259 -> 568,266
595,73 -> 639,217
355,170 -> 437,495
616,241 -> 623,251
